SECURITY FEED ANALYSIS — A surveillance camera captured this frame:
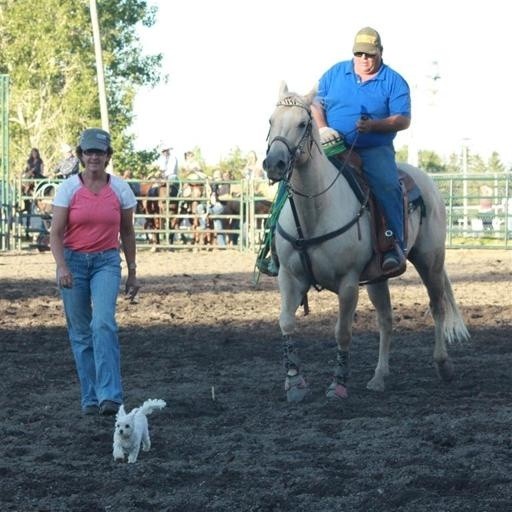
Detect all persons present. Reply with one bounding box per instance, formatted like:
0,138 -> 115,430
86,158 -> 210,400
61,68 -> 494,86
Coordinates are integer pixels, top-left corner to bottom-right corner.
26,147 -> 44,180
254,26 -> 410,276
54,144 -> 78,178
156,140 -> 179,192
210,170 -> 230,244
181,151 -> 204,196
51,129 -> 141,417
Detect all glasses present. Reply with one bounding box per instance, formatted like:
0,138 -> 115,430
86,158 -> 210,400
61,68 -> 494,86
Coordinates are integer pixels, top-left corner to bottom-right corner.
354,52 -> 375,57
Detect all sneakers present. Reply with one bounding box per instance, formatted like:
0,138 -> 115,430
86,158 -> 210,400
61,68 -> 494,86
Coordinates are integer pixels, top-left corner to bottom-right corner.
382,249 -> 399,271
84,400 -> 118,415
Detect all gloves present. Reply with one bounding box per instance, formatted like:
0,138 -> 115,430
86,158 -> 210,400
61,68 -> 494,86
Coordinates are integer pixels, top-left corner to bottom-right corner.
318,126 -> 344,147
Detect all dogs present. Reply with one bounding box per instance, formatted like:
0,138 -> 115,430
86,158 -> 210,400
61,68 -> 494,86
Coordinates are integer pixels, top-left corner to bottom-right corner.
111,397 -> 167,465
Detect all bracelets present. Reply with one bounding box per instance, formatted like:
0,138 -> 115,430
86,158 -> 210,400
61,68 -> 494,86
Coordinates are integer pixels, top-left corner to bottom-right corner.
128,262 -> 136,270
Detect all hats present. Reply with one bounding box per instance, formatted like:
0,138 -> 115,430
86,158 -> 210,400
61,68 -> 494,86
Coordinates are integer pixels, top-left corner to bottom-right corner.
80,128 -> 111,151
353,27 -> 381,55
160,144 -> 174,153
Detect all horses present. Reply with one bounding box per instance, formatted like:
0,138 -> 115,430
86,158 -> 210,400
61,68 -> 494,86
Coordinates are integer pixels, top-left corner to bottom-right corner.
17,168 -> 275,254
260,83 -> 473,407
